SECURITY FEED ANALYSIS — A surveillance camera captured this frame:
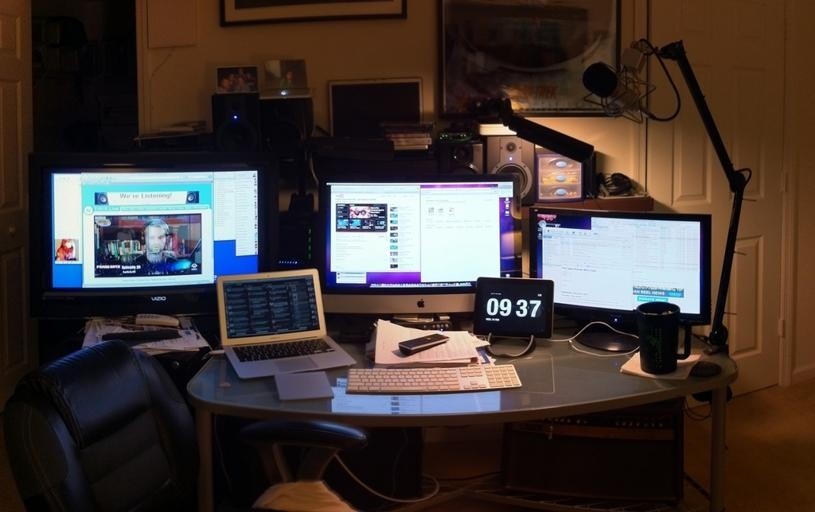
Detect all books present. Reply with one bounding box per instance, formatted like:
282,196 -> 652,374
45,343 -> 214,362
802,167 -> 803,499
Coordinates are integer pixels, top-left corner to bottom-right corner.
376,317 -> 491,370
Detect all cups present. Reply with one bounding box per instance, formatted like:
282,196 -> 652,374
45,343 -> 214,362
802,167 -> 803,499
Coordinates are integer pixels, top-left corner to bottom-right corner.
638,302 -> 692,377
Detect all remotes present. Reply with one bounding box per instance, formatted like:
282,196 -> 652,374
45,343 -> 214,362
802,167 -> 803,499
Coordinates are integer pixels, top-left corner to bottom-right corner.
398,333 -> 450,356
136,313 -> 180,326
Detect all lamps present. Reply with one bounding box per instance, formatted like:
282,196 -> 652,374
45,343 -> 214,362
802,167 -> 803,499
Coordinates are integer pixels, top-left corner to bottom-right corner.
462,91 -> 595,164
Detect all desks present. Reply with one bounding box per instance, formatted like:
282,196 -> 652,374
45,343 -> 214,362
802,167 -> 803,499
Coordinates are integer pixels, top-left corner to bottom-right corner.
186,329 -> 738,512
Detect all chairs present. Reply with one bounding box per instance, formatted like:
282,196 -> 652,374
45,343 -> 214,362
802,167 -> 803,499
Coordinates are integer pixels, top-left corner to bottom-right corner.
2,339 -> 283,512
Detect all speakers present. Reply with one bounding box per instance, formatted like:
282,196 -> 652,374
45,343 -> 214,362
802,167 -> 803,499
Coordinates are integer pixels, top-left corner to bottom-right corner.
437,139 -> 484,172
211,92 -> 261,149
261,97 -> 314,163
486,134 -> 537,206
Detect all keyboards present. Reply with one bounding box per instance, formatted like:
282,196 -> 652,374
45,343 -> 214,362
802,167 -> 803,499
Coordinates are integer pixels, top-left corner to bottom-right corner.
345,364 -> 523,395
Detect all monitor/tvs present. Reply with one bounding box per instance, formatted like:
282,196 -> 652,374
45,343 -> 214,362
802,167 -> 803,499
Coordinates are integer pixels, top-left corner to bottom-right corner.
528,206 -> 712,352
26,149 -> 281,318
328,76 -> 424,139
315,173 -> 522,323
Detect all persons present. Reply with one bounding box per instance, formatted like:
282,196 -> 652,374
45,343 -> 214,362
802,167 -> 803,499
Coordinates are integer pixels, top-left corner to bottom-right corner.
125,219 -> 187,277
221,69 -> 254,91
360,210 -> 370,218
280,70 -> 295,92
56,241 -> 76,262
350,207 -> 359,218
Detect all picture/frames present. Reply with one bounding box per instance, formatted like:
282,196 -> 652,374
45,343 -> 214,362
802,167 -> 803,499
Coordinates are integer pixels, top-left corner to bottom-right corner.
436,1 -> 622,120
219,0 -> 409,27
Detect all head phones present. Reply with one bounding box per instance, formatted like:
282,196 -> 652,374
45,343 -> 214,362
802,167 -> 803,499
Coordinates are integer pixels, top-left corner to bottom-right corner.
595,173 -> 632,197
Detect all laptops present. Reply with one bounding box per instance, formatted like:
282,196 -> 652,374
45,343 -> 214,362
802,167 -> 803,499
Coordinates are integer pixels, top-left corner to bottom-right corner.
216,268 -> 358,380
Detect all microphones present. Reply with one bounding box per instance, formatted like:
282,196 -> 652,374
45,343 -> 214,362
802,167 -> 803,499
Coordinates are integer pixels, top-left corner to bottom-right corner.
582,61 -> 642,111
